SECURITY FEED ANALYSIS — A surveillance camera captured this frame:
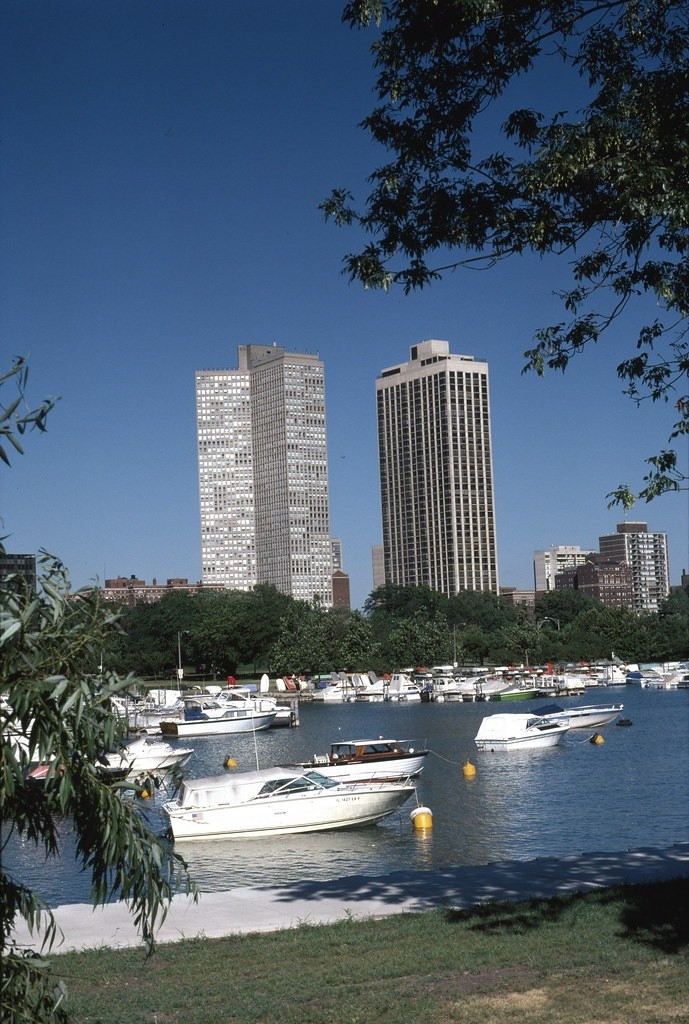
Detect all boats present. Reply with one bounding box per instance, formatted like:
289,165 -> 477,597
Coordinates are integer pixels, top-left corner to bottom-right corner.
107,683 -> 293,739
93,740 -> 199,771
162,737 -> 429,841
475,713 -> 571,753
529,704 -> 623,729
304,660 -> 689,702
